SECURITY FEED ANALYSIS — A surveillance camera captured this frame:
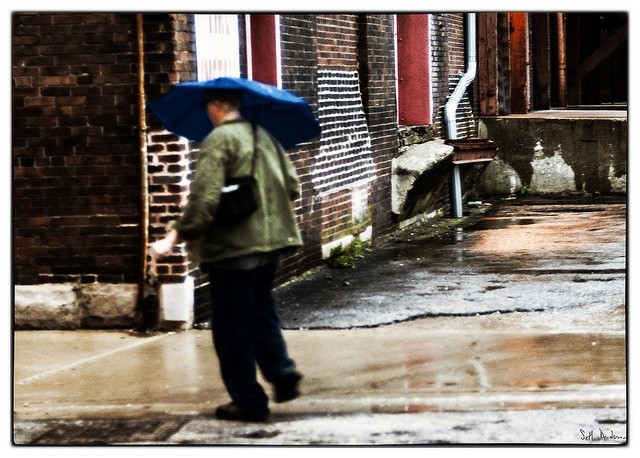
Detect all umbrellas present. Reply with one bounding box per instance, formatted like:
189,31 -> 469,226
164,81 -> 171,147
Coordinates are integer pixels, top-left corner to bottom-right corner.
156,77 -> 321,149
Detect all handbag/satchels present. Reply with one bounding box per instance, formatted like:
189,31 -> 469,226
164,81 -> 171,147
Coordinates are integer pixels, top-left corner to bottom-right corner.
216,177 -> 258,218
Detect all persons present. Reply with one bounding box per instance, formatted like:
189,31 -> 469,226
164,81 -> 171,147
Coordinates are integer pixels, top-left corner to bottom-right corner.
148,95 -> 303,421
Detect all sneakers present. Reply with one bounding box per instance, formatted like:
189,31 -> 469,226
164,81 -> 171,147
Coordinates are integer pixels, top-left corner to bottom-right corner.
275,372 -> 300,403
216,402 -> 271,422
